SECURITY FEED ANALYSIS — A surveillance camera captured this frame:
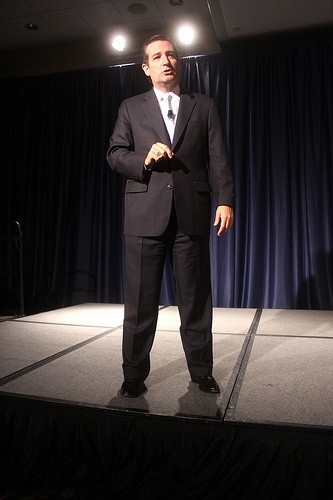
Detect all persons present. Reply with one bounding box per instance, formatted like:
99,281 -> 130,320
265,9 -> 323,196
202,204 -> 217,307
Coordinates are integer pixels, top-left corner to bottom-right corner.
105,34 -> 234,396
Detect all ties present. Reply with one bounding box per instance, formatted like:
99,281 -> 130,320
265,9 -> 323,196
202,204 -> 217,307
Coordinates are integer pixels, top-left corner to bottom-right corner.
161,93 -> 175,145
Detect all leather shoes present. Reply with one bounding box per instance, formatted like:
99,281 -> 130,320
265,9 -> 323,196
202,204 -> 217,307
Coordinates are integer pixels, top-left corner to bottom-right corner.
120,378 -> 144,397
191,374 -> 221,393
